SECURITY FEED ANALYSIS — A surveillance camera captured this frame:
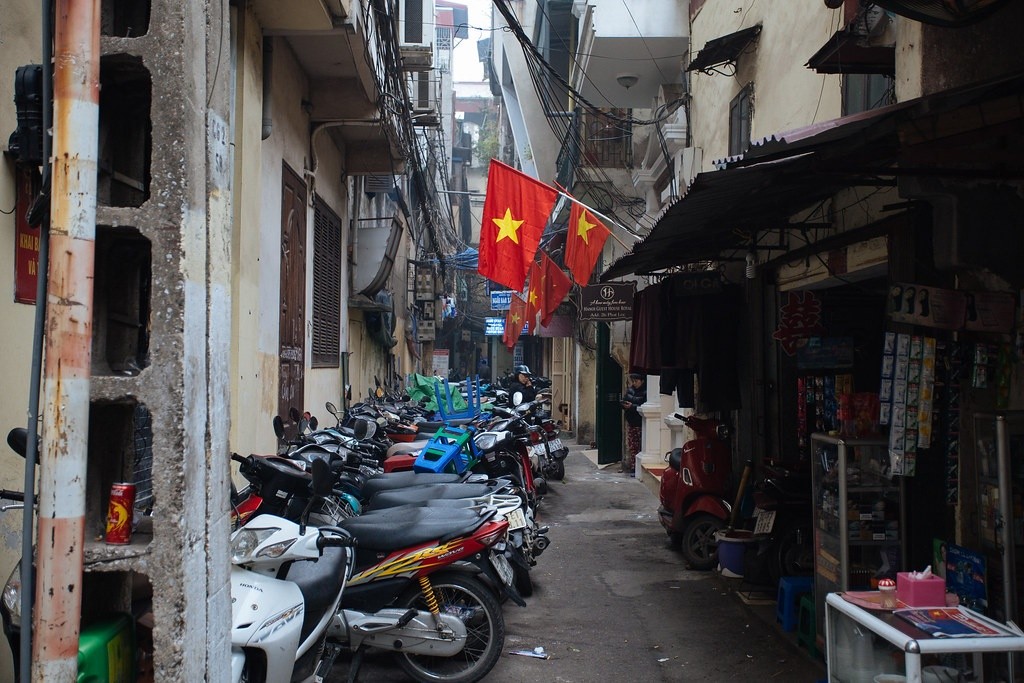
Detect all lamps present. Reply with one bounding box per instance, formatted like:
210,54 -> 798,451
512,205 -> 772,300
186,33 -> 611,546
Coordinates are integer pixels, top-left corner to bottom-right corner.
616,73 -> 639,91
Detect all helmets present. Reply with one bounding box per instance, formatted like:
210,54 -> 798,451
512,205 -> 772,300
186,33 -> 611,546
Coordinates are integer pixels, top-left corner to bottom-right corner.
515,364 -> 532,375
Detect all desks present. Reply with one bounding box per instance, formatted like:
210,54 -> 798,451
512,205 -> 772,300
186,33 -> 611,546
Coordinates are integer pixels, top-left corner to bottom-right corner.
825,588 -> 1024,683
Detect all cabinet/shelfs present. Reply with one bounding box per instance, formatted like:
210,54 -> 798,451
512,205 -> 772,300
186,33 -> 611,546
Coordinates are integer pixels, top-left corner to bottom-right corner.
811,433 -> 906,658
972,411 -> 1024,683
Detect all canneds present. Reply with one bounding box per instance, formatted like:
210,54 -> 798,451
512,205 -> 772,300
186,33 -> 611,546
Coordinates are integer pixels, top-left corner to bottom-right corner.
104,483 -> 136,545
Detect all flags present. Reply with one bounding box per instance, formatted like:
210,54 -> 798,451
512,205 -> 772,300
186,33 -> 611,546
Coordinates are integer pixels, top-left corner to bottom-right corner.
478,162 -> 559,294
525,262 -> 542,336
503,293 -> 527,352
564,201 -> 610,288
539,251 -> 572,328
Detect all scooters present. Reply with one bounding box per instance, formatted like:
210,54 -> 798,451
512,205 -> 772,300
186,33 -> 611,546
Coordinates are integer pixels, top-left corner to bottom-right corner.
231,366 -> 571,683
0,427 -> 357,683
742,456 -> 814,607
656,412 -> 734,570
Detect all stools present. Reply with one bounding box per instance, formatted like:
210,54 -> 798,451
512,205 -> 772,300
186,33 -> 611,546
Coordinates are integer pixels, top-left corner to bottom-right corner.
776,574 -> 818,658
414,423 -> 483,478
434,374 -> 480,419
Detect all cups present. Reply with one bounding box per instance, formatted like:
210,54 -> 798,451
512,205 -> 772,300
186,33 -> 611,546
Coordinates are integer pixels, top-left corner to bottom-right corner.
834,615 -> 896,683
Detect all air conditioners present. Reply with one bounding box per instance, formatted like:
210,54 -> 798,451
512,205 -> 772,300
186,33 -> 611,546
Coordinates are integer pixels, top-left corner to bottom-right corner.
398,0 -> 435,68
412,71 -> 438,125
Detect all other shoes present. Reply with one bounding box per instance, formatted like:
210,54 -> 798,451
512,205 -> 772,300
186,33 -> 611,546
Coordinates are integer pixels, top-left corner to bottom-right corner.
631,472 -> 635,478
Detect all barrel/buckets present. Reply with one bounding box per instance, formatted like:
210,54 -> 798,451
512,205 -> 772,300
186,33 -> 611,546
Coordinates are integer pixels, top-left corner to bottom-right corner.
716,530 -> 760,572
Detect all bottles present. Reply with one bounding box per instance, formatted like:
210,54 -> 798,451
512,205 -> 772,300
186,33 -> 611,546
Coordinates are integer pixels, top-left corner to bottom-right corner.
878,579 -> 896,609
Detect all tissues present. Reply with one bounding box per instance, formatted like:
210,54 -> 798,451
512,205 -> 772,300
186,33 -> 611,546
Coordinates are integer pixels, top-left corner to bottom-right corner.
896,565 -> 947,609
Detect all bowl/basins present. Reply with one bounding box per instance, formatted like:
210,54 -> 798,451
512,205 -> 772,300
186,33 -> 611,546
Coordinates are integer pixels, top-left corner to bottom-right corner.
874,665 -> 959,683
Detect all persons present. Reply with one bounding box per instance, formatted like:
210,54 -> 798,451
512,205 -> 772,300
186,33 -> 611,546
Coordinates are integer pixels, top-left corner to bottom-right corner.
889,286 -> 929,317
479,359 -> 490,379
936,544 -> 947,576
508,364 -> 536,425
622,372 -> 647,476
130,520 -> 154,658
